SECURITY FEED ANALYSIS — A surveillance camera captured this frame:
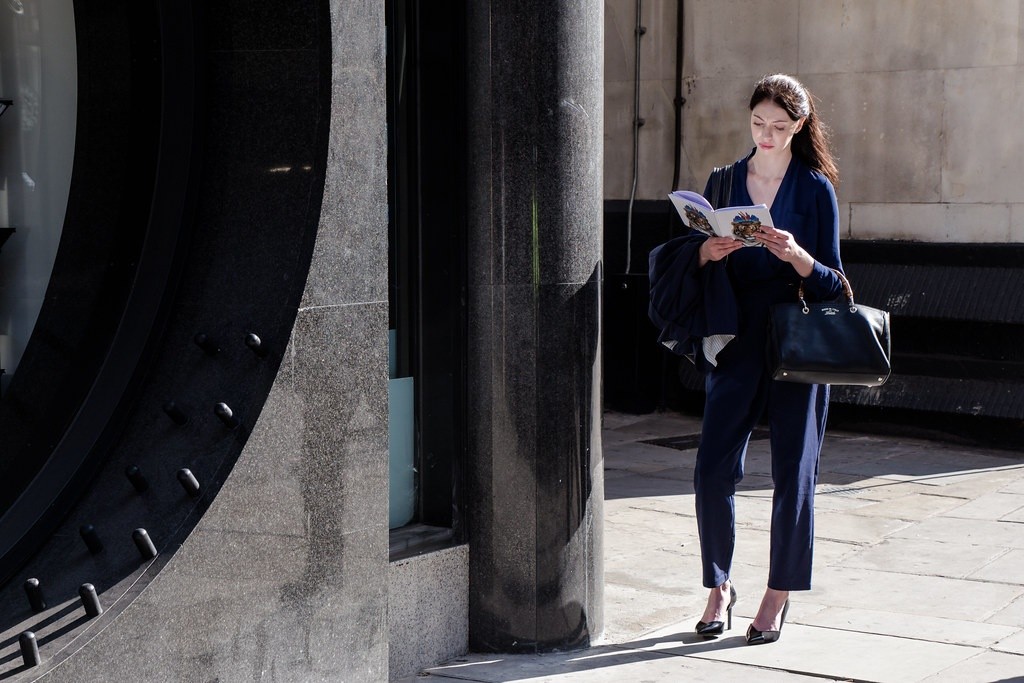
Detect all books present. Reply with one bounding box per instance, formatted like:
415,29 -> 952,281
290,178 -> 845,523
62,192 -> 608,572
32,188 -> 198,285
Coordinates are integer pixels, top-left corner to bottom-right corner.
668,190 -> 776,247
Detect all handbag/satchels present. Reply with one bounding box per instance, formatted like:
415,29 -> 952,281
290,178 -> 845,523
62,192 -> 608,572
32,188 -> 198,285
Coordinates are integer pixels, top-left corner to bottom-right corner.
768,271 -> 891,386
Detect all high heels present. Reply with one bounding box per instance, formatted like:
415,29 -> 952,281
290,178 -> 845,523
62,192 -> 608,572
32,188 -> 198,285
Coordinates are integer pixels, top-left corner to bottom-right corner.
747,584 -> 789,645
696,579 -> 737,637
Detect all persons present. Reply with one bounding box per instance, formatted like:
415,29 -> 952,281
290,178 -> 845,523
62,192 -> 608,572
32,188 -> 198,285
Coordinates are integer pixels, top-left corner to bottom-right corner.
664,73 -> 850,648
